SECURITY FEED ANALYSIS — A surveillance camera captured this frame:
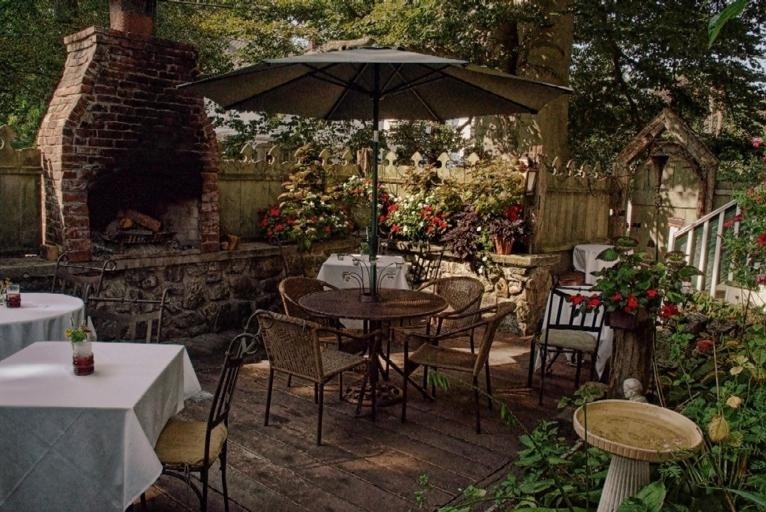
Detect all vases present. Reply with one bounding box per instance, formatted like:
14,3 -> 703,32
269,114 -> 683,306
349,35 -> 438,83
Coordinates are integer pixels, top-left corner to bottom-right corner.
608,309 -> 636,331
72,336 -> 95,375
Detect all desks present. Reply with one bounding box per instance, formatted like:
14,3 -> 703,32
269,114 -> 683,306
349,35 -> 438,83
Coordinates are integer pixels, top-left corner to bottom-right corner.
0,281 -> 96,363
319,253 -> 411,290
294,284 -> 447,417
0,338 -> 216,512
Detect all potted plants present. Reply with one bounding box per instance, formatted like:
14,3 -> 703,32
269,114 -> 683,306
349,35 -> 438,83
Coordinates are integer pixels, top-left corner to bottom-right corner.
492,217 -> 525,253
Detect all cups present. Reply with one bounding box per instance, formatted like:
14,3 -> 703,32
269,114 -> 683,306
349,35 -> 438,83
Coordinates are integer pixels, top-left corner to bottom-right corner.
71,331 -> 94,376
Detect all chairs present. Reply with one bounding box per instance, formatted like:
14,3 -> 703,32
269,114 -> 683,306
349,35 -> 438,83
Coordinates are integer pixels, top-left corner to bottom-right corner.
397,299 -> 516,432
277,273 -> 372,402
81,282 -> 175,344
525,286 -> 606,408
252,307 -> 369,446
139,309 -> 274,512
384,275 -> 484,396
49,250 -> 116,315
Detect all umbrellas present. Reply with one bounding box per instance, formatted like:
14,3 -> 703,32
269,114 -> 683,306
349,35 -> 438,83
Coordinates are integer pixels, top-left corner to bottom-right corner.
176,48 -> 575,298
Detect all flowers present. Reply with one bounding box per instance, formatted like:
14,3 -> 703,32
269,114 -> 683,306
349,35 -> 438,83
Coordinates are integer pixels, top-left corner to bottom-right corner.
566,235 -> 706,317
253,179 -> 453,244
64,317 -> 92,340
503,205 -> 518,223
